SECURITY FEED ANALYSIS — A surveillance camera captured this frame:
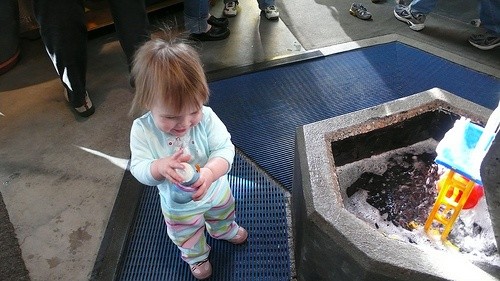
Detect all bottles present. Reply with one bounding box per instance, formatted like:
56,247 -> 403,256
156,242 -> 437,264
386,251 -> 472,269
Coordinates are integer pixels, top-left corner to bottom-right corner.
176,162 -> 199,186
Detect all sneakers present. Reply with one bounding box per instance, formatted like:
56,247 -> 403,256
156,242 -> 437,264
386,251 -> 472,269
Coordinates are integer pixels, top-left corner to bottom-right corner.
223,1 -> 239,16
265,4 -> 280,21
468,30 -> 500,50
349,4 -> 372,19
394,4 -> 426,31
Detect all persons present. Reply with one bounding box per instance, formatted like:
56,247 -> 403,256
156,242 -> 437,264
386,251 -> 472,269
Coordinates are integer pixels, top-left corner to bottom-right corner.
394,0 -> 500,50
130,38 -> 248,279
184,0 -> 230,41
33,0 -> 151,118
224,0 -> 280,20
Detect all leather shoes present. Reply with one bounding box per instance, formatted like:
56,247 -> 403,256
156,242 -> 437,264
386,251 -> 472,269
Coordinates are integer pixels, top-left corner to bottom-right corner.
207,15 -> 228,27
189,25 -> 231,41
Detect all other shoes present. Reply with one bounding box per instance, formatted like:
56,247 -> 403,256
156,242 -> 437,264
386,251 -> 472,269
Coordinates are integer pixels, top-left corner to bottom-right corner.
228,226 -> 247,243
190,260 -> 211,280
64,88 -> 95,117
